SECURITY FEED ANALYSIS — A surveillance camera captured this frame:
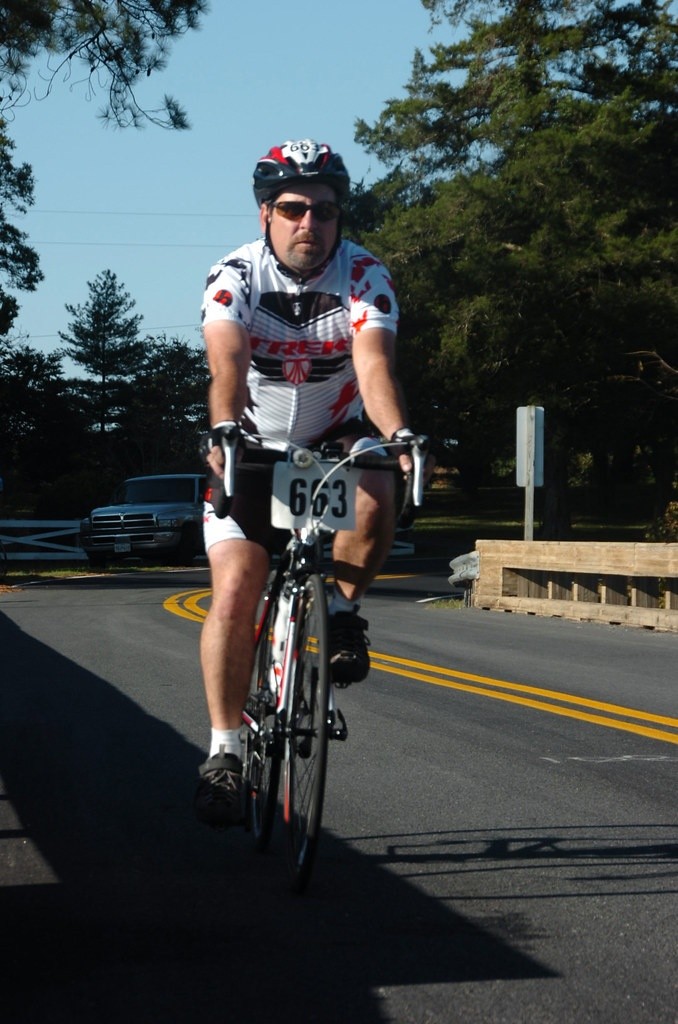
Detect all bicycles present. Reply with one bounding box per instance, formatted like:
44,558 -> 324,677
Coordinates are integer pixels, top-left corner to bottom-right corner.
202,422 -> 429,895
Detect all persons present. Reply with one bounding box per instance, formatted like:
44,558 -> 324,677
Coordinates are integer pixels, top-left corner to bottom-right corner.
192,138 -> 438,831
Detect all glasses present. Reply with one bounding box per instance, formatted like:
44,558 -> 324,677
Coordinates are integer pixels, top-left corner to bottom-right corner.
271,200 -> 340,222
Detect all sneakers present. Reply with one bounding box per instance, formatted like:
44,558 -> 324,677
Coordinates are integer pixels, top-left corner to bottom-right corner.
194,744 -> 243,825
327,604 -> 372,683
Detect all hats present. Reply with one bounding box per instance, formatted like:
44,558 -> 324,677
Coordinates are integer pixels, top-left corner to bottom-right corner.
254,139 -> 350,209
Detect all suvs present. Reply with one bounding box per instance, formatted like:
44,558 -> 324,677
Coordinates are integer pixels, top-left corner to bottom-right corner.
79,472 -> 207,566
